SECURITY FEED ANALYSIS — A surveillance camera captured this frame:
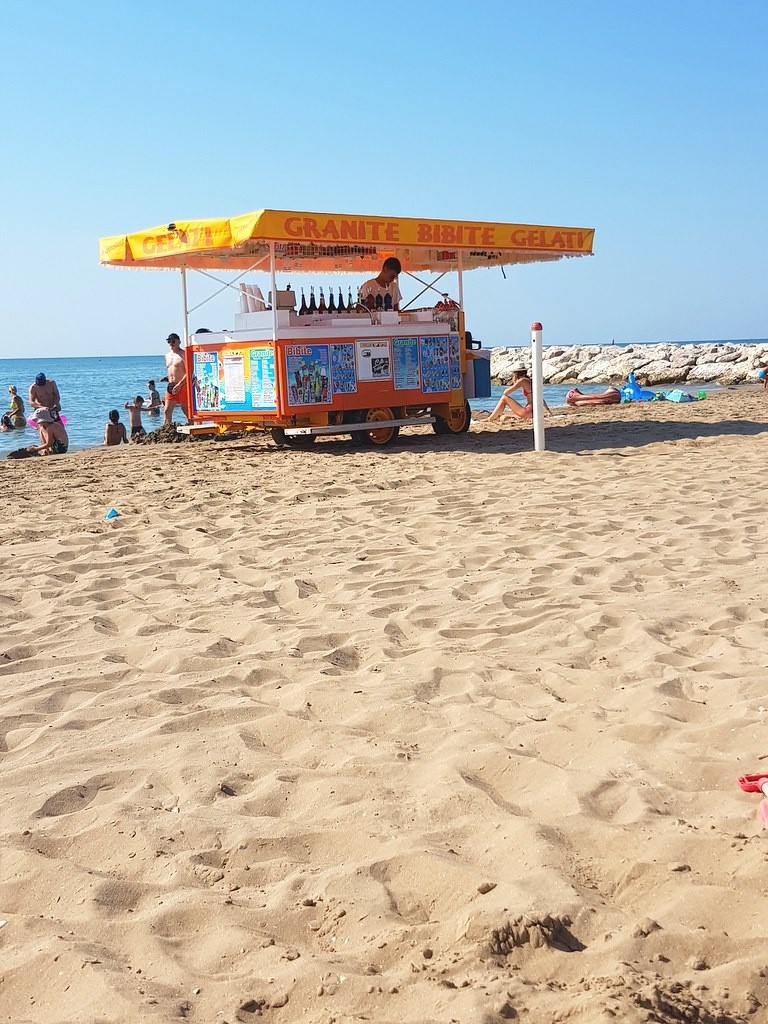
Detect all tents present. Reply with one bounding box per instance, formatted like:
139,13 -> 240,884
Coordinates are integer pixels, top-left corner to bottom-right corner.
96,209 -> 595,344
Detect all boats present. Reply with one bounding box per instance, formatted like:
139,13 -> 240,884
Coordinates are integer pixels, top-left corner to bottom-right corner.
566,386 -> 622,407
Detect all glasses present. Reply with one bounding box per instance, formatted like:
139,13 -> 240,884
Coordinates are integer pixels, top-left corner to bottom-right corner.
389,270 -> 398,279
168,340 -> 179,343
8,390 -> 12,393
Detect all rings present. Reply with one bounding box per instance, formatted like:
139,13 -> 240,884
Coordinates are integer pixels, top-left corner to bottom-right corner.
174,389 -> 176,391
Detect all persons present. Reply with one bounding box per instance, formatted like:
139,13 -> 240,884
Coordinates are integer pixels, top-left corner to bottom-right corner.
0,385 -> 27,433
25,372 -> 69,456
358,257 -> 403,311
757,366 -> 768,390
164,332 -> 188,423
104,379 -> 165,445
479,362 -> 553,423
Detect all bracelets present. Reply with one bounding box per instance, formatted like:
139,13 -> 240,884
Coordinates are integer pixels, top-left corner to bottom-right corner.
546,408 -> 549,410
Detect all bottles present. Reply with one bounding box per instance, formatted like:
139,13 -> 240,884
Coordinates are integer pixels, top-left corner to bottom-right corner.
337,287 -> 347,314
309,286 -> 319,314
299,287 -> 310,315
328,287 -> 338,314
317,286 -> 329,314
291,360 -> 331,404
197,383 -> 218,407
356,287 -> 366,313
384,284 -> 392,311
367,287 -> 375,313
375,287 -> 383,311
347,286 -> 354,313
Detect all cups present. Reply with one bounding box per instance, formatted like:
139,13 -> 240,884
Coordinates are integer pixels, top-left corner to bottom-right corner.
239,283 -> 266,313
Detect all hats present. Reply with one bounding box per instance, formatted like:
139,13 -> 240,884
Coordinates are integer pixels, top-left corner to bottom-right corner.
31,408 -> 54,424
166,333 -> 180,340
510,361 -> 529,372
34,372 -> 46,386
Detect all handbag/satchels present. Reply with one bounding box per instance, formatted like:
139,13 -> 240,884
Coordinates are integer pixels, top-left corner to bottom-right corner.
167,384 -> 174,394
